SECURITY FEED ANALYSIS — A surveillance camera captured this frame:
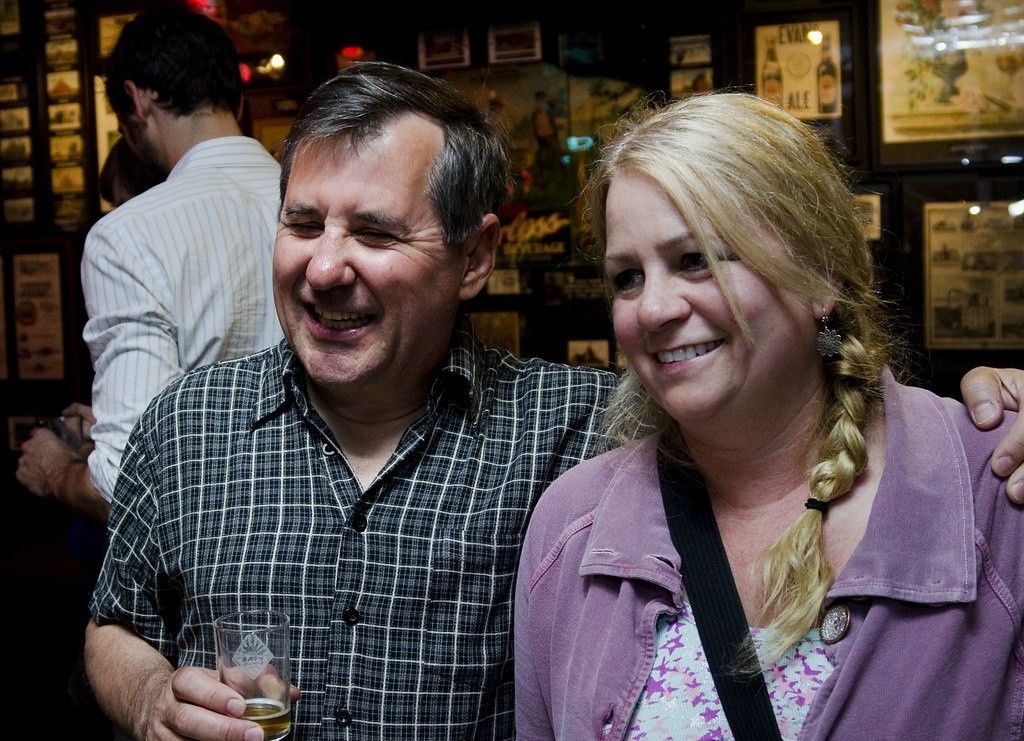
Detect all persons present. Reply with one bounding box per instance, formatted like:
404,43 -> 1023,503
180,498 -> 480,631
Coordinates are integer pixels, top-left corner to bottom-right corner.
80,61 -> 1024,741
15,4 -> 282,520
513,89 -> 1024,741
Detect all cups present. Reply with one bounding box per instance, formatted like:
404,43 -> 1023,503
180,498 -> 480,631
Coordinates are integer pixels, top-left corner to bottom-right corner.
212,609 -> 292,740
35,415 -> 82,452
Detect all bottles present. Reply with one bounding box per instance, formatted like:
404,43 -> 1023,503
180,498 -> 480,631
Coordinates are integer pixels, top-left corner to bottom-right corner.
762,35 -> 784,110
817,33 -> 839,115
